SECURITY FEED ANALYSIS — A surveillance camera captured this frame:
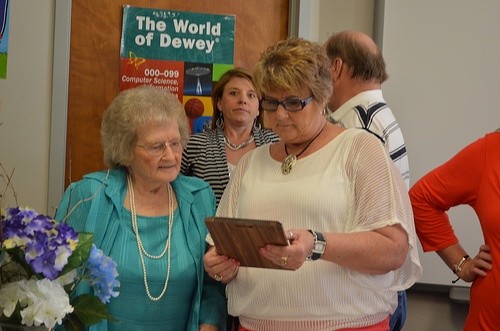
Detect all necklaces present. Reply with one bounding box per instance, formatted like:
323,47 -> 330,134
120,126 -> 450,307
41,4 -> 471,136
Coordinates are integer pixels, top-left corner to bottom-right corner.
127,173 -> 174,301
281,123 -> 325,175
224,135 -> 255,150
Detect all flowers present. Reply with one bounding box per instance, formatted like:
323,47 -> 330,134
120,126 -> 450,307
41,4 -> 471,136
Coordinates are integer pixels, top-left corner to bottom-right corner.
0,204 -> 122,331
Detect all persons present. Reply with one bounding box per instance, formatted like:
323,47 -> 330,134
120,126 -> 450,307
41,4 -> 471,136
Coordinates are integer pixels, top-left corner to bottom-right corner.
203,36 -> 422,331
322,29 -> 411,331
408,132 -> 500,331
53,84 -> 227,331
180,67 -> 281,213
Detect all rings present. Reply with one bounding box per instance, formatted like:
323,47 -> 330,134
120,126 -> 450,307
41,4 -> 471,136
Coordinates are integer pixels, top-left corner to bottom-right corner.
281,256 -> 288,266
214,274 -> 222,281
289,230 -> 293,239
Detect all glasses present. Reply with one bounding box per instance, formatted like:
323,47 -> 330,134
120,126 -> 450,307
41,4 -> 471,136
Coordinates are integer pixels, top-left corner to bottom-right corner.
261,95 -> 315,111
134,136 -> 186,156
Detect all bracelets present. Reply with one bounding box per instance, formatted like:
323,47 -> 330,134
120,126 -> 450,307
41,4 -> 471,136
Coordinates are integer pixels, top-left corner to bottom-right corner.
452,255 -> 469,284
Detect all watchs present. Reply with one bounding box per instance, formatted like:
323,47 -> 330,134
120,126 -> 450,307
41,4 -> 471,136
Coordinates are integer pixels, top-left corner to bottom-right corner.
305,229 -> 326,261
